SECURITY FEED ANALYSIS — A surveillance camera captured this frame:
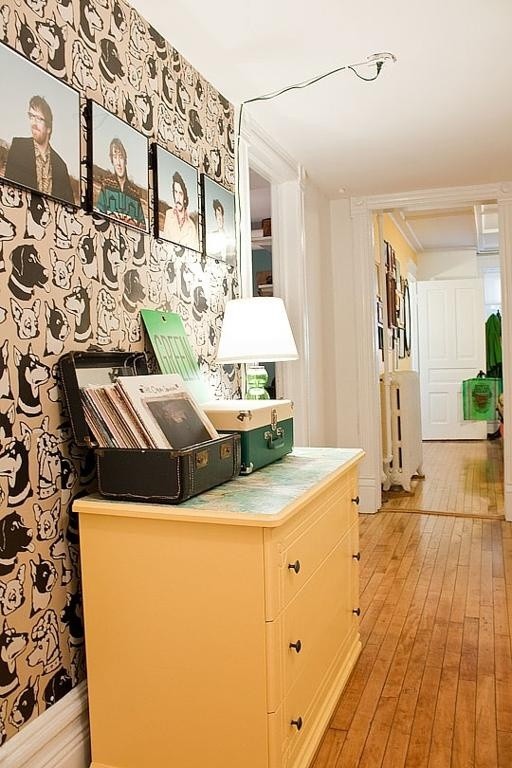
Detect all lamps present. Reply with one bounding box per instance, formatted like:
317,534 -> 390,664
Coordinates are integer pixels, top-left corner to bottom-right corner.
215,297 -> 300,400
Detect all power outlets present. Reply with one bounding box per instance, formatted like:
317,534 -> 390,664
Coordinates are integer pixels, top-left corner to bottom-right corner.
365,51 -> 396,70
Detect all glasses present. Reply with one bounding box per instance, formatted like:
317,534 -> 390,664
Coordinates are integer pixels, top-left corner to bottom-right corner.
29,112 -> 48,122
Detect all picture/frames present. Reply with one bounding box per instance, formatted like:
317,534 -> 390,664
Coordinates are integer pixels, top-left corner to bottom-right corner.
200,172 -> 236,267
87,98 -> 150,236
151,142 -> 200,254
376,239 -> 411,361
0,38 -> 82,210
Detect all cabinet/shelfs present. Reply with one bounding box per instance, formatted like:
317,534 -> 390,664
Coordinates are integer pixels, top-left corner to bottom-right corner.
251,236 -> 273,297
71,445 -> 367,768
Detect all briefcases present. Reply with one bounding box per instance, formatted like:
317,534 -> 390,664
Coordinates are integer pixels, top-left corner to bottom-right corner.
197,399 -> 294,476
58,351 -> 242,507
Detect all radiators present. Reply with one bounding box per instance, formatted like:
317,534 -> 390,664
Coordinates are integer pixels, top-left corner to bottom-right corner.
380,371 -> 425,494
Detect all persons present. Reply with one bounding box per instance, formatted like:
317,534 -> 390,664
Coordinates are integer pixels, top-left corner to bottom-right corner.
206,199 -> 236,266
163,172 -> 199,251
96,138 -> 146,231
4,96 -> 75,205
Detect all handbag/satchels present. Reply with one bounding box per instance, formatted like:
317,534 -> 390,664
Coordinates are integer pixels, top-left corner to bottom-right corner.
461,371 -> 503,421
